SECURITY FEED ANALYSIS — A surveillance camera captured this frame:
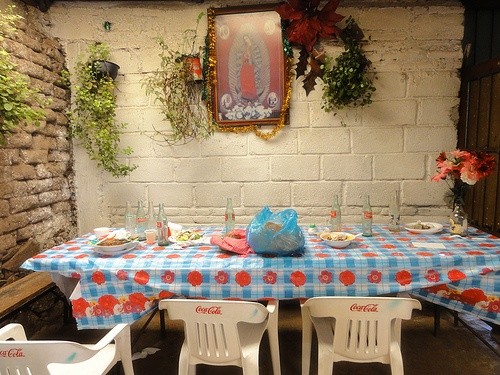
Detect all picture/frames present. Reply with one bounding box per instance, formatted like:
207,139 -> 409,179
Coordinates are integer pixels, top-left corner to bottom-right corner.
207,4 -> 291,126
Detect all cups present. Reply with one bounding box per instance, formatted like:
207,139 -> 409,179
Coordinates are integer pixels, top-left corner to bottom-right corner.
145,229 -> 156,244
94,227 -> 109,239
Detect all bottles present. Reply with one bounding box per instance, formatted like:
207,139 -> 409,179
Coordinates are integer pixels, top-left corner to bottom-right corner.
361,195 -> 373,237
225,197 -> 235,232
157,203 -> 168,246
388,190 -> 401,232
136,201 -> 147,237
125,201 -> 135,238
147,200 -> 156,229
330,194 -> 341,232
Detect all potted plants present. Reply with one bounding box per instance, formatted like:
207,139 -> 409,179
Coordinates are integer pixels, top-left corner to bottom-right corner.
140,11 -> 213,147
319,43 -> 379,130
61,38 -> 141,177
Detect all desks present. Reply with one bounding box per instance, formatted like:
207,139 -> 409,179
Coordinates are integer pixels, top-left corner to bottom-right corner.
20,224 -> 500,375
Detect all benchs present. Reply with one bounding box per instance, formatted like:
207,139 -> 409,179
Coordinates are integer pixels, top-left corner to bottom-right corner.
0,271 -> 71,324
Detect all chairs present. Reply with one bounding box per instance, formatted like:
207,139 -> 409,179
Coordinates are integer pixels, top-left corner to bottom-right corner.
300,294 -> 422,375
0,323 -> 134,375
158,298 -> 281,375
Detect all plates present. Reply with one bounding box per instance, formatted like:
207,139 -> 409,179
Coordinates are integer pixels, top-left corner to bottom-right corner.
93,243 -> 136,255
168,235 -> 206,244
404,222 -> 443,234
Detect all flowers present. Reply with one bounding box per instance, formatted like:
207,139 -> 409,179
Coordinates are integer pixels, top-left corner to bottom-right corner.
275,0 -> 346,96
430,148 -> 496,204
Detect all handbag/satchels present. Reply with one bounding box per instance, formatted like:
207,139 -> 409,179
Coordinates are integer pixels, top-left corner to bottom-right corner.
245,205 -> 305,254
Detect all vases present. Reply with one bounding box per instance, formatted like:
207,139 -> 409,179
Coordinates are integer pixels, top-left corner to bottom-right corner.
452,198 -> 465,226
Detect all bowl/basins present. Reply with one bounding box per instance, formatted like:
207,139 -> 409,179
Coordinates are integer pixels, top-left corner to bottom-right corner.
320,232 -> 355,248
95,240 -> 131,251
